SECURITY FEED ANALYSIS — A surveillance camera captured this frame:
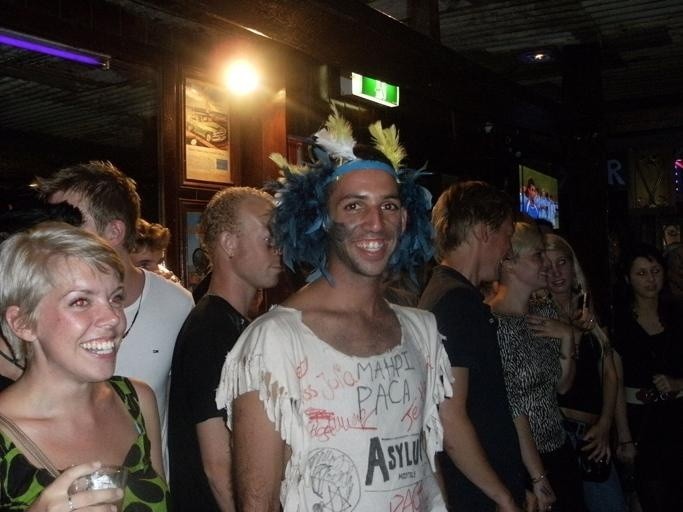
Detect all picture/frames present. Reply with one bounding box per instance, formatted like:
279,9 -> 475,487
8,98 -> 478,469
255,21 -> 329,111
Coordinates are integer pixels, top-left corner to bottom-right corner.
175,62 -> 262,326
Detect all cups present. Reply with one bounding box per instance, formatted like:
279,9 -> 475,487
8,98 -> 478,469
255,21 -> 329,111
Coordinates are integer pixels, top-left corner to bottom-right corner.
63,464 -> 128,512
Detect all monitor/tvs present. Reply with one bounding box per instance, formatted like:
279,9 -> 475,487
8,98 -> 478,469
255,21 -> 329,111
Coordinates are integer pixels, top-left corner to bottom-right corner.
518,162 -> 561,231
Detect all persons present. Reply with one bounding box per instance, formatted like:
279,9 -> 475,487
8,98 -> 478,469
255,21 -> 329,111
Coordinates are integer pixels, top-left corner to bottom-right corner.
167,186 -> 297,512
611,245 -> 680,512
130,218 -> 179,283
484,223 -> 630,512
1,222 -> 168,512
520,177 -> 559,226
419,179 -> 537,512
34,161 -> 197,489
212,97 -> 516,511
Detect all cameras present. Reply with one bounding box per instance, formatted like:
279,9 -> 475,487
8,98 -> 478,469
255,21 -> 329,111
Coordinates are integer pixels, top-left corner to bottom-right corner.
637,371 -> 675,404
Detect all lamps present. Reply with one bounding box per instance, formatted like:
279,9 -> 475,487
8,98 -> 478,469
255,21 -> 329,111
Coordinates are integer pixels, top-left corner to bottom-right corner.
0,30 -> 111,72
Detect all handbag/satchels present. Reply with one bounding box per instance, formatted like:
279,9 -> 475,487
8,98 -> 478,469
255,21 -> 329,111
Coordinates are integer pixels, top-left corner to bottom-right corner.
575,439 -> 611,481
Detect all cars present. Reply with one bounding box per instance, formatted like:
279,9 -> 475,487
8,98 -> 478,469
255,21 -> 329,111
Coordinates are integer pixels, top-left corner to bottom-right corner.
185,110 -> 228,144
